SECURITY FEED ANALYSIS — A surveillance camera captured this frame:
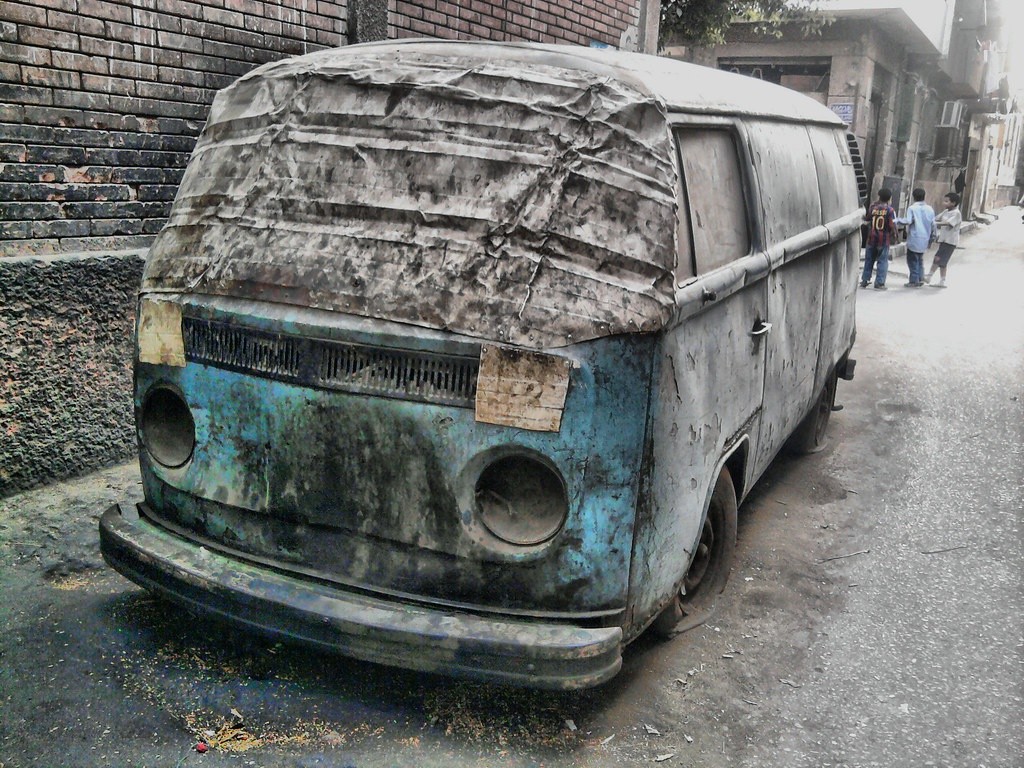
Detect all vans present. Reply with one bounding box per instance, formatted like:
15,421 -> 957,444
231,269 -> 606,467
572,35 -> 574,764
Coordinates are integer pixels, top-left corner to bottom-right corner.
98,37 -> 869,692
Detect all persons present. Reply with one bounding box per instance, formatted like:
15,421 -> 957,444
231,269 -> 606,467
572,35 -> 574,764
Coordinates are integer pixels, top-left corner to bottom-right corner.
893,188 -> 937,287
923,192 -> 962,288
860,188 -> 898,291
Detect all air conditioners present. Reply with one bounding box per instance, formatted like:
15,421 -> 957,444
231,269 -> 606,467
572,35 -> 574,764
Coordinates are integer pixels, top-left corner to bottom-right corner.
940,100 -> 963,128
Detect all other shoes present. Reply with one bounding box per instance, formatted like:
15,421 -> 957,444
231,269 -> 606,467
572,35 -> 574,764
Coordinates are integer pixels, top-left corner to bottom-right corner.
860,281 -> 867,289
923,271 -> 948,288
873,283 -> 888,291
904,279 -> 924,288
